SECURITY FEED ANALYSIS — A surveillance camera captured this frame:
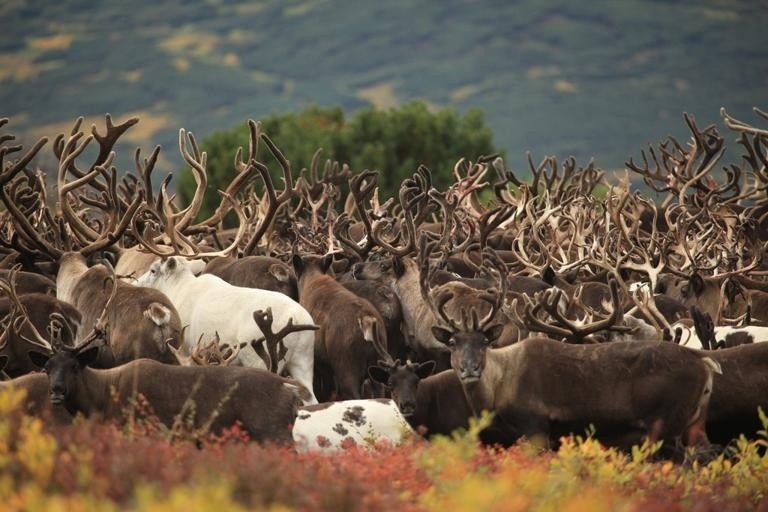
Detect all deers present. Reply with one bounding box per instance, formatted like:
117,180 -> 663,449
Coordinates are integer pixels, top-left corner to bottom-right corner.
0,103 -> 768,463
